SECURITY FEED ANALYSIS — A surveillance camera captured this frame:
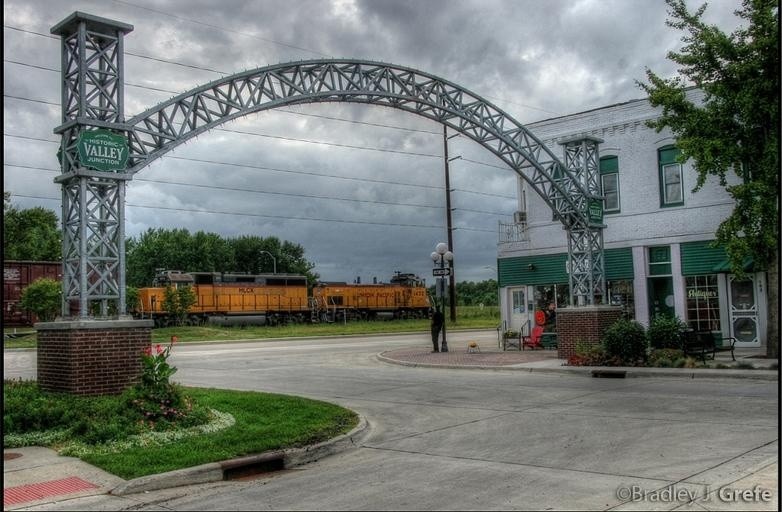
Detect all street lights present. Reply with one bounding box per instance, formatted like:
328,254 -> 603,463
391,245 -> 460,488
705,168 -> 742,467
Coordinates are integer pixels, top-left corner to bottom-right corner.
259,249 -> 277,275
429,241 -> 455,351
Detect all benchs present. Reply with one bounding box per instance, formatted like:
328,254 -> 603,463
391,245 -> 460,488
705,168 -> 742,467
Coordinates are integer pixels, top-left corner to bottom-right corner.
679,333 -> 736,364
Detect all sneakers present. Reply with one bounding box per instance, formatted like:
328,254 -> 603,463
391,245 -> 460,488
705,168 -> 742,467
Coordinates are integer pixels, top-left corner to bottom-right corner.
432,350 -> 439,353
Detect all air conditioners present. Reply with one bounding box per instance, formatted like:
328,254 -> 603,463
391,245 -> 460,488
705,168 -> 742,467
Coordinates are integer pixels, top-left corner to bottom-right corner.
513,210 -> 527,224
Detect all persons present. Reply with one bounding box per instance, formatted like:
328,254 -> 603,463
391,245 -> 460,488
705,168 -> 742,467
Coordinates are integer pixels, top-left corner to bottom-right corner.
430,304 -> 444,353
542,303 -> 556,333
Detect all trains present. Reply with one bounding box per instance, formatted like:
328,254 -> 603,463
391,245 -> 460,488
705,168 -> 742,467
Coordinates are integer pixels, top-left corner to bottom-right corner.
4,259 -> 119,330
135,266 -> 439,326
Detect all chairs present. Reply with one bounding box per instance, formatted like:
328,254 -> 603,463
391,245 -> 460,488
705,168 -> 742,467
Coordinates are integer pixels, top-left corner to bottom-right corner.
522,326 -> 544,350
